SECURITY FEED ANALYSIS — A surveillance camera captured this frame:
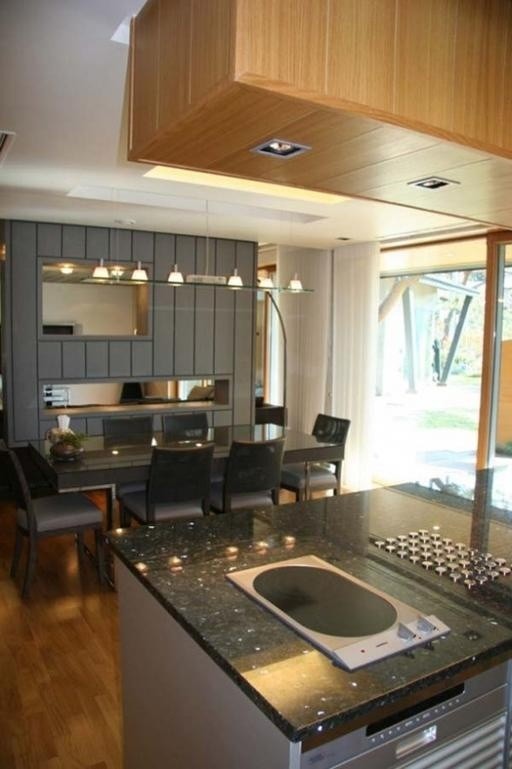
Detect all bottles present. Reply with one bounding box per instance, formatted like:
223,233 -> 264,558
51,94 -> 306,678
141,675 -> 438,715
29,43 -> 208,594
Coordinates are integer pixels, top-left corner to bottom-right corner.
255,379 -> 265,405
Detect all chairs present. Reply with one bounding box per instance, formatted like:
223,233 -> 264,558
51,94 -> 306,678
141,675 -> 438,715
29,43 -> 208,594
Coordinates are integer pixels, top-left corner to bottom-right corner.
210,435 -> 289,517
102,415 -> 154,436
117,441 -> 218,531
161,412 -> 211,433
279,412 -> 349,503
0,448 -> 110,601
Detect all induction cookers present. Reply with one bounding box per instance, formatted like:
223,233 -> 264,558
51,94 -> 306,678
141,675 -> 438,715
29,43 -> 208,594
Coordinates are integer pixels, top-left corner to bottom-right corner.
225,551 -> 450,671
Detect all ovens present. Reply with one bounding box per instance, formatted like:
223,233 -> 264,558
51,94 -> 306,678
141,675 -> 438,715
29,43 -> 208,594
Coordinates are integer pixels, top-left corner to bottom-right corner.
302,642 -> 511,769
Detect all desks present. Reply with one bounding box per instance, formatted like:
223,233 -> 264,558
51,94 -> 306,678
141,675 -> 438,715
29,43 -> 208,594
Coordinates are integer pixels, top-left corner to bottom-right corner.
27,422 -> 346,530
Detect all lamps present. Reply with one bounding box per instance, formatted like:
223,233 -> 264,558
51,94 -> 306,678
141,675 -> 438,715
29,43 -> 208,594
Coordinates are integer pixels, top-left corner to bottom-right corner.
43,256 -> 316,295
